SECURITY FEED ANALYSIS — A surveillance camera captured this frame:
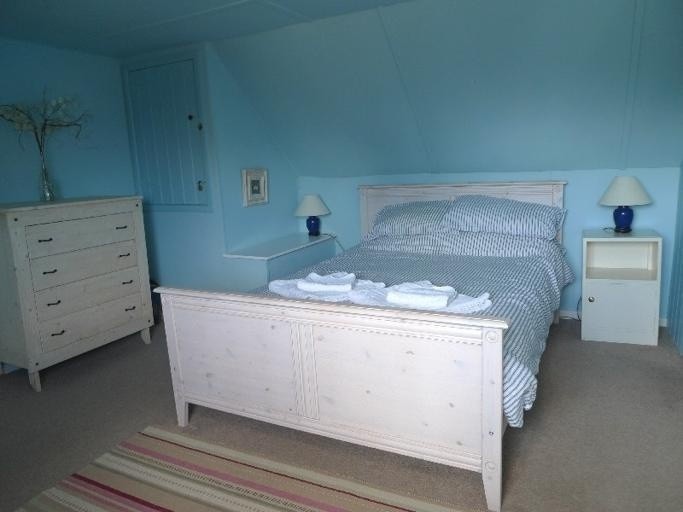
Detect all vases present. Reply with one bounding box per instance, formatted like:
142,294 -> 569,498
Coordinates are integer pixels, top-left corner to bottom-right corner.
38,151 -> 56,202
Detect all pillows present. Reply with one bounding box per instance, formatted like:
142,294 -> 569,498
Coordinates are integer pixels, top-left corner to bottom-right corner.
363,200 -> 450,239
441,195 -> 567,240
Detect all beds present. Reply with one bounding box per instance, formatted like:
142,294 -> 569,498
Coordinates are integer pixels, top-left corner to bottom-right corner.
153,180 -> 573,512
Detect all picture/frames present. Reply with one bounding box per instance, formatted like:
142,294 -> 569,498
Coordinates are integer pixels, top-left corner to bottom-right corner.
242,168 -> 269,207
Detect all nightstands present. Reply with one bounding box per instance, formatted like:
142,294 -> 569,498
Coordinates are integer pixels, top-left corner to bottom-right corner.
581,230 -> 662,346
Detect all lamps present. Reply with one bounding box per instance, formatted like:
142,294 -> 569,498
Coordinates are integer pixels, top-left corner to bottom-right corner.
294,194 -> 330,236
597,175 -> 653,233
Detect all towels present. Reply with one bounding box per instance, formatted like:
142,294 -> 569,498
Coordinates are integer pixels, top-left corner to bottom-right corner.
269,271 -> 492,314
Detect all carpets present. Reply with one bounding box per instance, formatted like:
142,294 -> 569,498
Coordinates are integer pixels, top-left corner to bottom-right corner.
12,425 -> 466,512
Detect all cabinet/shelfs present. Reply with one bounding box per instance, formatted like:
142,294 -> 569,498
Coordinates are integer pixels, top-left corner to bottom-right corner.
0,195 -> 156,393
